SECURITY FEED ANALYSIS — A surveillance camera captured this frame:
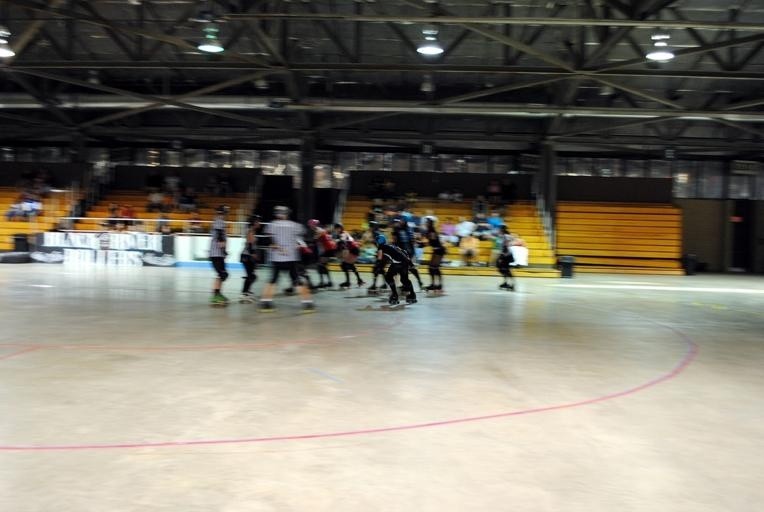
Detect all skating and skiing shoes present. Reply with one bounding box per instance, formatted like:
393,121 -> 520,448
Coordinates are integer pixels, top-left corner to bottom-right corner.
499,282 -> 514,289
208,279 -> 442,311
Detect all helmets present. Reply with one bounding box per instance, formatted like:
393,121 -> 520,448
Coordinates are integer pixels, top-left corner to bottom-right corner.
496,224 -> 508,230
214,202 -> 436,246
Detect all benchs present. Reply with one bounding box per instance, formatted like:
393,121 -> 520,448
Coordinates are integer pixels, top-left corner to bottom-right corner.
323,196 -> 685,278
76,181 -> 257,252
